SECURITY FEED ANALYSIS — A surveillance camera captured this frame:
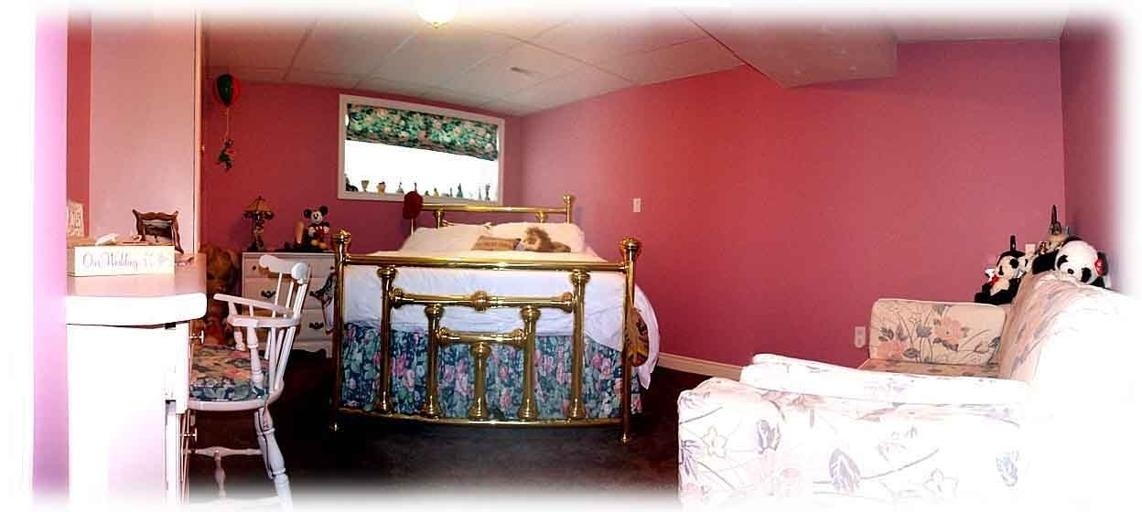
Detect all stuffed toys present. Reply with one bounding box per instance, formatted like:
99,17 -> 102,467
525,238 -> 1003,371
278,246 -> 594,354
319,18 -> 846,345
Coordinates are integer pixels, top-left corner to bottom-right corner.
973,231 -> 1108,306
191,242 -> 243,350
303,205 -> 332,249
520,226 -> 572,253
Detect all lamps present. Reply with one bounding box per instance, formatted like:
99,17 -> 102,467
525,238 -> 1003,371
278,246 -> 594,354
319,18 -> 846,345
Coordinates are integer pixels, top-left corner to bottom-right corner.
240,194 -> 275,252
208,71 -> 241,170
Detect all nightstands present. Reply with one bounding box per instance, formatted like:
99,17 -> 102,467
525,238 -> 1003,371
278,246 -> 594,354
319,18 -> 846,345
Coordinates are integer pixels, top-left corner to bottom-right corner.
241,250 -> 338,360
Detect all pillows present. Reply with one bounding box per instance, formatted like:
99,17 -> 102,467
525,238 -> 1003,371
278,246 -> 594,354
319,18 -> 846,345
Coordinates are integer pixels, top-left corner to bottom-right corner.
740,352 -> 1027,408
400,221 -> 585,254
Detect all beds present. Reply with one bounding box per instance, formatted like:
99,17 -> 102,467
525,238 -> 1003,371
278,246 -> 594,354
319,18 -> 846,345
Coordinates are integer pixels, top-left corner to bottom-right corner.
329,191 -> 642,445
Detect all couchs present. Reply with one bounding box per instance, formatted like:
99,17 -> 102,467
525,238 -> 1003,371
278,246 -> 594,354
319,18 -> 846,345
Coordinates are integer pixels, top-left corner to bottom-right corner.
677,270 -> 1131,506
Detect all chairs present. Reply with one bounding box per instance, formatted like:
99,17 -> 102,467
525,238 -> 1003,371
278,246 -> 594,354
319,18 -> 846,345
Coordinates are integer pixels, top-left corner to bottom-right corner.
180,255 -> 312,502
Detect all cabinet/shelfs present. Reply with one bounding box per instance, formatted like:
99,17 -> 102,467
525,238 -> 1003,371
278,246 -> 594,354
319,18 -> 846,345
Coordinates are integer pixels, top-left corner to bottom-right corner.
66,251 -> 208,503
